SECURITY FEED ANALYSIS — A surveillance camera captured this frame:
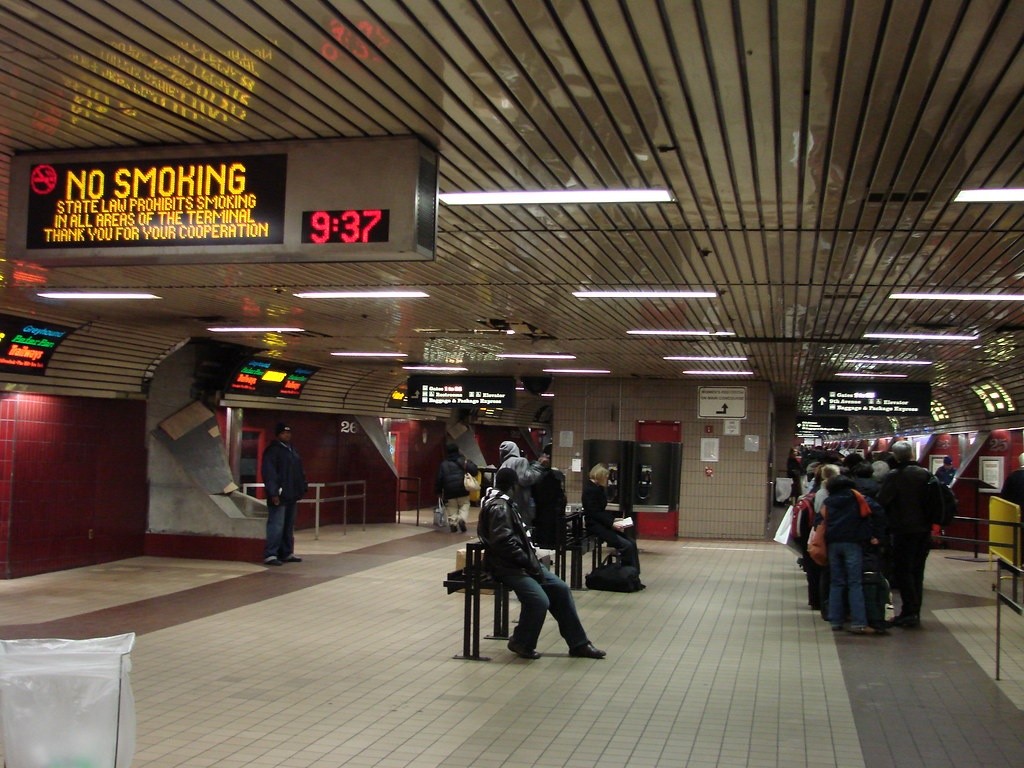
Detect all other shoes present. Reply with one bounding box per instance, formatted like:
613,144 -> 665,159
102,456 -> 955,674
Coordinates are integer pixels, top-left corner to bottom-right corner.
264,559 -> 283,566
507,636 -> 541,658
893,613 -> 921,626
850,626 -> 880,634
450,523 -> 457,532
459,519 -> 467,532
281,554 -> 302,562
569,641 -> 606,657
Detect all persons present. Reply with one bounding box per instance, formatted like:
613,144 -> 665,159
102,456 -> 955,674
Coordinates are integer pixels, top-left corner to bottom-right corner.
582,463 -> 637,566
259,421 -> 309,566
435,440 -> 479,532
477,468 -> 606,659
786,440 -> 958,635
493,440 -> 568,549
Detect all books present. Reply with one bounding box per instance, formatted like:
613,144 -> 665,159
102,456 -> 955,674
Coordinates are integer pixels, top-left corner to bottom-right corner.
613,517 -> 634,530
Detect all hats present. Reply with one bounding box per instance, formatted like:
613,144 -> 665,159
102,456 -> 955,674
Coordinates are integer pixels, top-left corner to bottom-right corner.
274,421 -> 293,436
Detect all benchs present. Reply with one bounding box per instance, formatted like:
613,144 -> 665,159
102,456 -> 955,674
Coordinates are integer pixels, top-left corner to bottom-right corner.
444,510 -> 603,661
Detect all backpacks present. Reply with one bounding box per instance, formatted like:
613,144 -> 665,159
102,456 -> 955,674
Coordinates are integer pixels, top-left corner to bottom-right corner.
790,491 -> 819,541
926,472 -> 957,526
862,570 -> 894,629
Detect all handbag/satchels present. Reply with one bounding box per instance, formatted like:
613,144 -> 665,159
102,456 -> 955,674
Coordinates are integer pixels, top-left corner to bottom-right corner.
584,552 -> 637,593
932,524 -> 943,544
809,519 -> 827,564
464,472 -> 480,492
